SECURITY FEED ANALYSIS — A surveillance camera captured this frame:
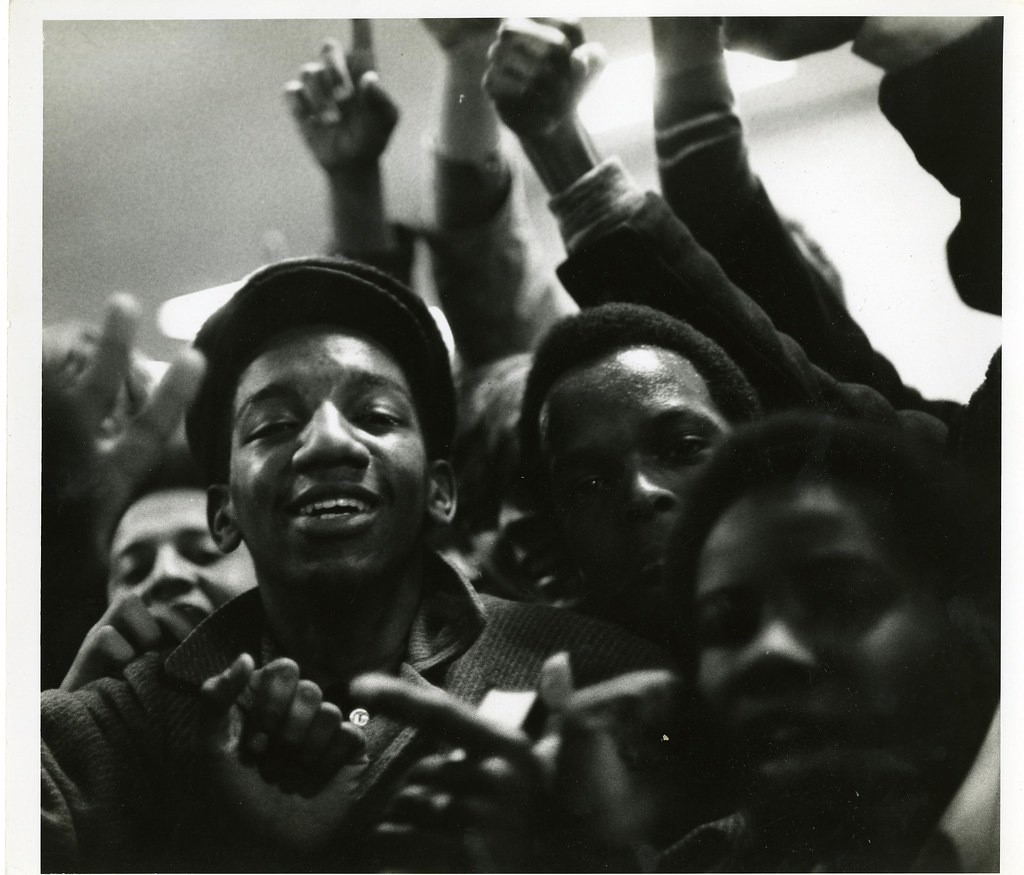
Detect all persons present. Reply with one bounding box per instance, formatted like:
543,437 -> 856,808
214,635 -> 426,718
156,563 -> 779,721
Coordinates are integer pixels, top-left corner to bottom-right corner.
43,16 -> 1004,874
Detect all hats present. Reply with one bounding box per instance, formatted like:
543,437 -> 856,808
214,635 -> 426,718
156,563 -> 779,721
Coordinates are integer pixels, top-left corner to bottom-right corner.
186,257 -> 459,484
